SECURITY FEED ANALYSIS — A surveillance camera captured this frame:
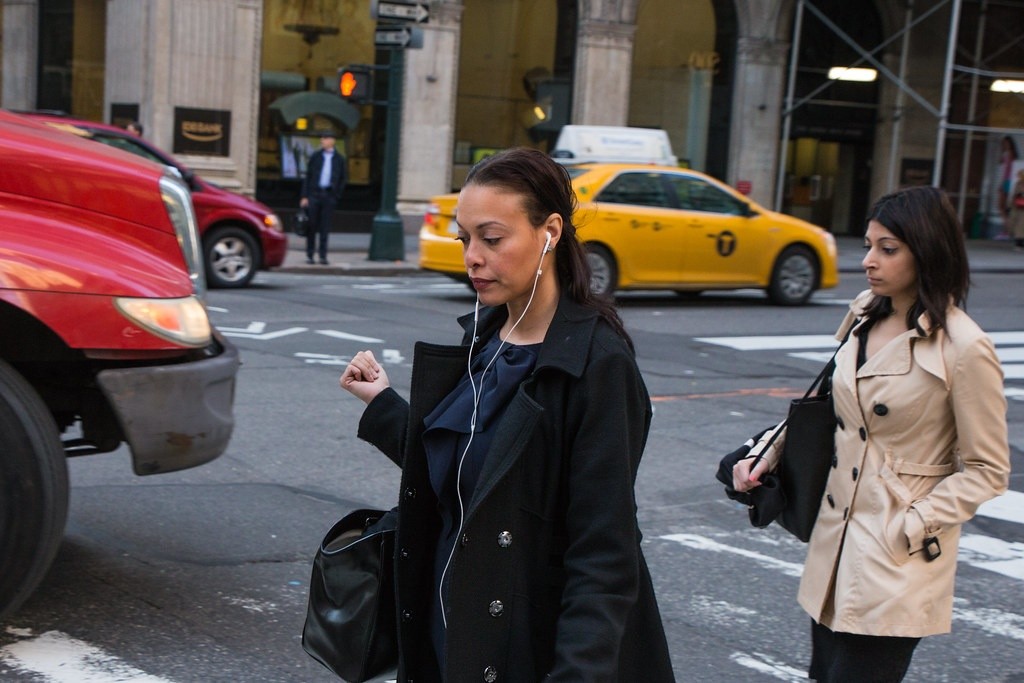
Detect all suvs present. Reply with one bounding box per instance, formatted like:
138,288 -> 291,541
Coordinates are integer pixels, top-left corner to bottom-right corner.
8,109 -> 287,288
0,106 -> 238,623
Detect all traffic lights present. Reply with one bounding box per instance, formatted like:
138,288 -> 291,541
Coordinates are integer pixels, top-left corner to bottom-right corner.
337,67 -> 374,102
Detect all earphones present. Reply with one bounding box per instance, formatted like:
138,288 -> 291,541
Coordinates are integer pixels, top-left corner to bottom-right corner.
543,231 -> 552,255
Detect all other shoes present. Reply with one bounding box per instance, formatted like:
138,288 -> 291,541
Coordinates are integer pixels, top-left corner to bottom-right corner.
305,255 -> 316,266
318,256 -> 329,264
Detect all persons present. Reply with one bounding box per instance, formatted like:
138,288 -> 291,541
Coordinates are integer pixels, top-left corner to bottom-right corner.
978,136 -> 1024,224
339,148 -> 676,683
127,121 -> 143,138
735,188 -> 1011,683
299,130 -> 345,264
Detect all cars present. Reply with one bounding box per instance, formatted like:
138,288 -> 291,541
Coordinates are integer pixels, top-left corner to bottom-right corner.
419,125 -> 841,305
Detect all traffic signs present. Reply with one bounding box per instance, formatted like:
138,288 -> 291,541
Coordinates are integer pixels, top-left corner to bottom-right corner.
374,26 -> 413,47
371,0 -> 430,24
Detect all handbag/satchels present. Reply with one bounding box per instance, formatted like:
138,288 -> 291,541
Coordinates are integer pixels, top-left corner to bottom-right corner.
773,383 -> 838,543
716,426 -> 790,530
293,210 -> 309,236
300,505 -> 402,683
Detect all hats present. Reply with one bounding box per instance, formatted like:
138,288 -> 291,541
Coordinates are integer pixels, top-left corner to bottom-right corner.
316,130 -> 338,140
